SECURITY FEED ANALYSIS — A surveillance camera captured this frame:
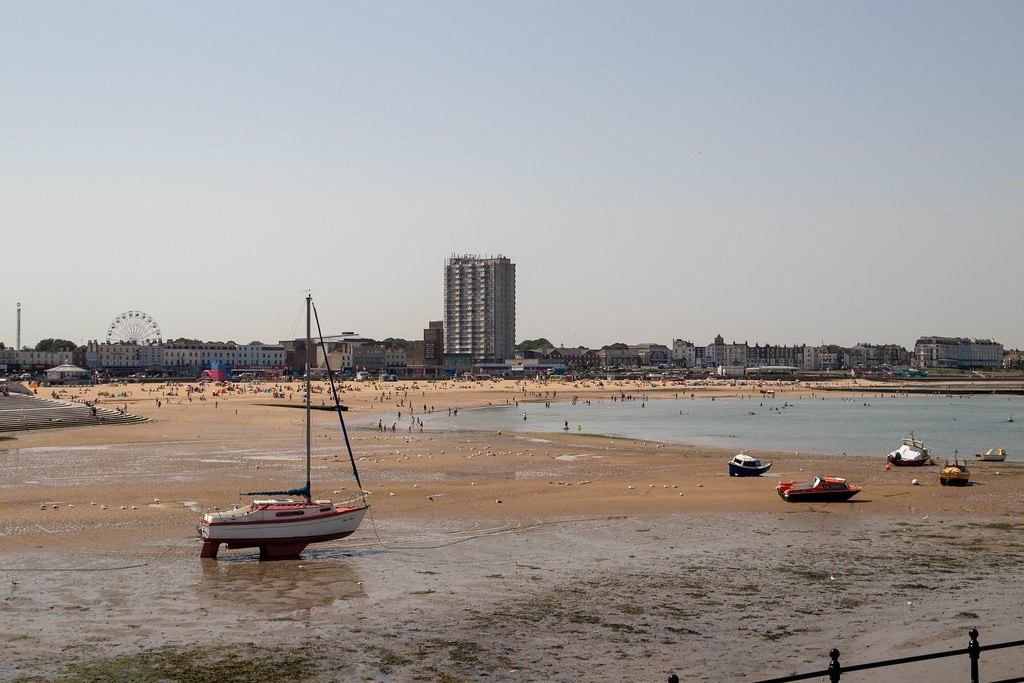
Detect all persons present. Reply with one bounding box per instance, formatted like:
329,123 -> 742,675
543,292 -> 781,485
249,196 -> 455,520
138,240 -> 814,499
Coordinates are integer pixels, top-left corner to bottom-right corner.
0,380 -> 132,422
149,372 -> 1014,434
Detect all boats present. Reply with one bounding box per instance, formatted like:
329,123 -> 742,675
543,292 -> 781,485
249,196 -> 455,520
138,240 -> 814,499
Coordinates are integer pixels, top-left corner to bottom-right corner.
939,448 -> 970,486
776,474 -> 863,503
886,429 -> 932,467
728,453 -> 773,477
975,448 -> 1008,461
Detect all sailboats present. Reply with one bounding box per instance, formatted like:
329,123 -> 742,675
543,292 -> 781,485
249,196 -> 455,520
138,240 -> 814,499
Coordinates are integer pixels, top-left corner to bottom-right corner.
195,288 -> 372,561
